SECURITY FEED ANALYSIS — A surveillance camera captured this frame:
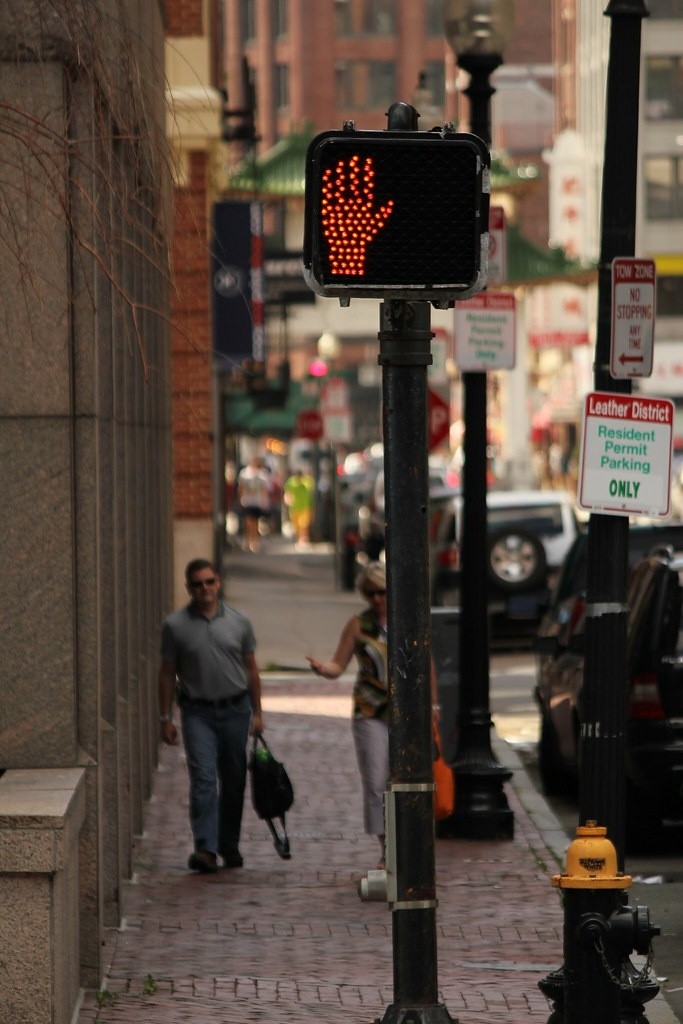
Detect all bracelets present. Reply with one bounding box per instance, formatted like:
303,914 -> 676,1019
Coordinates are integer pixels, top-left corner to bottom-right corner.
431,702 -> 443,711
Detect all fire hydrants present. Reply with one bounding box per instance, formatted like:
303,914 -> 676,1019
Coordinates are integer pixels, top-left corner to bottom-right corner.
536,816 -> 669,1024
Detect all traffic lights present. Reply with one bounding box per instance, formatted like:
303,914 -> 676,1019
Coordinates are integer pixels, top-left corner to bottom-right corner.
301,125 -> 490,307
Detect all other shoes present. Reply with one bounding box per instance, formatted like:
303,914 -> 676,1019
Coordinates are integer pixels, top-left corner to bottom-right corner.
218,842 -> 244,866
378,859 -> 386,871
187,854 -> 218,872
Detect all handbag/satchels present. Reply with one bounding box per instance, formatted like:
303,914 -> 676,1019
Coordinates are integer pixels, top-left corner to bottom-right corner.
432,722 -> 454,820
252,731 -> 293,859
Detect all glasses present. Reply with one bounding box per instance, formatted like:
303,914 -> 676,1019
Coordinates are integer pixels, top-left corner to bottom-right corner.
190,576 -> 218,588
362,589 -> 386,596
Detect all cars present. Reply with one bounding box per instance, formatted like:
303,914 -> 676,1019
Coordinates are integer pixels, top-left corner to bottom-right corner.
322,441 -> 495,598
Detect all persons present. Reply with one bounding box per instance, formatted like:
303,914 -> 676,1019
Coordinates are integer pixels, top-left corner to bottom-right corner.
215,417 -> 330,564
305,562 -> 443,877
156,558 -> 267,870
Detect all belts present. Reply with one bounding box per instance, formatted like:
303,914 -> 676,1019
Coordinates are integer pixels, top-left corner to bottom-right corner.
179,690 -> 250,706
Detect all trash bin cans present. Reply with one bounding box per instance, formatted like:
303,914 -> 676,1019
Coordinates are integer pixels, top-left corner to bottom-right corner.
429,605 -> 461,766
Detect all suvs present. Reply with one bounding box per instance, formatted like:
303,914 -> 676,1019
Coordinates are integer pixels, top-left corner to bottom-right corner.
536,516 -> 681,797
615,542 -> 682,860
437,488 -> 590,647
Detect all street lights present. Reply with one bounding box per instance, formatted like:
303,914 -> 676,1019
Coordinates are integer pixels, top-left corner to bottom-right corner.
438,5 -> 520,845
